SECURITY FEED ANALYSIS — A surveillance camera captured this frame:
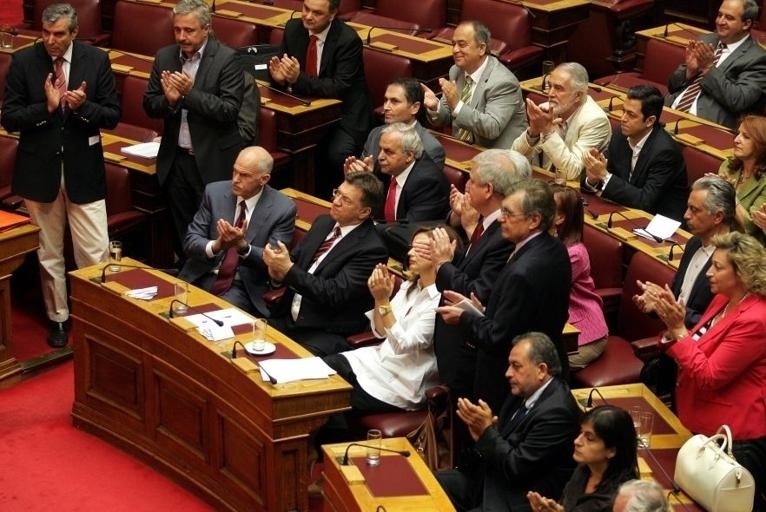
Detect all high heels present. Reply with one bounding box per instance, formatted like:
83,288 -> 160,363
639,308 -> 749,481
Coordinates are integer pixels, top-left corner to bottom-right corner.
308,478 -> 322,499
307,446 -> 319,479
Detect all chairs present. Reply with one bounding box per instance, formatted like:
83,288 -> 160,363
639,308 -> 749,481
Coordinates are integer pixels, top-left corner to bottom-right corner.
101,75 -> 168,146
594,42 -> 690,98
442,161 -> 464,193
208,17 -> 256,55
357,46 -> 410,122
110,1 -> 173,59
0,134 -> 20,201
269,27 -> 286,54
267,0 -> 355,24
582,223 -> 622,333
149,12 -> 255,57
434,0 -> 543,79
3,164 -> 145,312
265,280 -> 387,349
569,250 -> 682,389
349,327 -> 454,472
259,108 -> 288,186
30,0 -> 102,43
336,2 -> 443,43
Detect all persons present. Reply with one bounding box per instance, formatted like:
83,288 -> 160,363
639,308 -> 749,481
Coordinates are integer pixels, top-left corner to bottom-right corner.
262,171 -> 387,354
268,0 -> 370,174
580,84 -> 688,222
550,183 -> 609,369
750,202 -> 766,233
237,70 -> 260,146
509,61 -> 612,181
344,123 -> 449,258
436,179 -> 572,415
413,148 -> 529,390
611,478 -> 667,512
359,80 -> 445,171
646,234 -> 766,472
446,183 -> 482,242
418,20 -> 530,149
632,177 -> 737,397
704,115 -> 766,233
432,331 -> 579,512
142,1 -> 244,253
663,0 -> 766,129
526,405 -> 640,512
0,4 -> 120,348
177,146 -> 296,318
323,224 -> 464,429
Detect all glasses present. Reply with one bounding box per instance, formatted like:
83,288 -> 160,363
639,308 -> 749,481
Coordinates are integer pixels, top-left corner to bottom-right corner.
501,208 -> 527,218
332,188 -> 353,207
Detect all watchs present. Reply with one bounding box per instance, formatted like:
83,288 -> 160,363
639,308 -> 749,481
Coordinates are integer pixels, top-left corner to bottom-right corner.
675,335 -> 684,343
375,306 -> 391,317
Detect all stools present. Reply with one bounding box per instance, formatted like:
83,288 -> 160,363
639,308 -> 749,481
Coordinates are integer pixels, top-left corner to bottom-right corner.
597,0 -> 655,72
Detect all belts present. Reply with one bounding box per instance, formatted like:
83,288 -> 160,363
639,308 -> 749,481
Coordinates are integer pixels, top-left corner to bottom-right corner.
176,147 -> 195,157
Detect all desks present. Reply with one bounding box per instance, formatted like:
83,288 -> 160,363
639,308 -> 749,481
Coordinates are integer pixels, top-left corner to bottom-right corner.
570,383 -> 706,511
423,129 -> 695,274
519,76 -> 739,190
69,256 -> 351,511
501,0 -> 599,80
110,1 -> 453,107
274,188 -> 580,375
0,110 -> 168,250
318,437 -> 460,512
0,208 -> 41,388
636,24 -> 714,86
96,47 -> 344,197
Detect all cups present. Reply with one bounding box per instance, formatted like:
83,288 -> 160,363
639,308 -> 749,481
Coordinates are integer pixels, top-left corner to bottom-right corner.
175,282 -> 188,314
367,429 -> 382,465
638,412 -> 655,447
556,168 -> 568,186
628,406 -> 641,428
108,241 -> 122,272
252,319 -> 267,351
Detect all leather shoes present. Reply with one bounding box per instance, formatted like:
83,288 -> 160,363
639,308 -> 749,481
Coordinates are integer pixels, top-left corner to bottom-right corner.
47,320 -> 69,347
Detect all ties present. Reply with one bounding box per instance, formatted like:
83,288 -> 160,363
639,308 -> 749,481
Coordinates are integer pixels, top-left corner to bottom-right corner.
312,226 -> 340,262
471,219 -> 485,245
304,35 -> 318,80
208,201 -> 246,296
54,57 -> 67,113
500,401 -> 527,437
455,76 -> 472,144
674,42 -> 727,114
384,178 -> 398,222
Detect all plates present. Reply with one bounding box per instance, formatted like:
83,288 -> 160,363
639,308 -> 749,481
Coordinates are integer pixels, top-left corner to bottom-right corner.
243,341 -> 277,356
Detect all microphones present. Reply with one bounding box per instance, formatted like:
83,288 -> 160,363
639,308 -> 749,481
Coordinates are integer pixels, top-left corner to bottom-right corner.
368,25 -> 433,43
102,260 -> 179,283
675,114 -> 740,138
664,17 -> 701,40
639,435 -> 682,494
231,340 -> 278,388
169,299 -> 224,328
587,384 -> 609,409
667,240 -> 686,263
341,441 -> 413,466
372,217 -> 411,227
73,35 -> 98,45
256,79 -> 311,106
607,93 -> 626,110
542,72 -> 602,94
607,209 -> 662,247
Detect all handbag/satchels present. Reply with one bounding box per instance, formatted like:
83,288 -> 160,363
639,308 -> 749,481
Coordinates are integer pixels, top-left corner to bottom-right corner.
406,385 -> 456,473
673,423 -> 756,512
